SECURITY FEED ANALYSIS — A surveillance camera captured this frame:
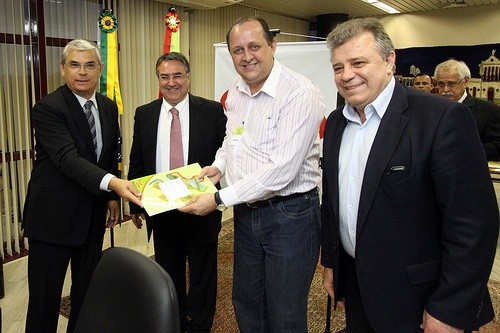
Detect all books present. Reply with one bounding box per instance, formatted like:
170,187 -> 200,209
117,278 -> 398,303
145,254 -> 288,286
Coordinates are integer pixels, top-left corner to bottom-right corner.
487,161 -> 500,183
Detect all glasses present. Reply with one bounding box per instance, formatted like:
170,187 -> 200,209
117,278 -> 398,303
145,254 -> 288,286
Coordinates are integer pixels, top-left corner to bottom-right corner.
436,78 -> 465,89
63,62 -> 103,72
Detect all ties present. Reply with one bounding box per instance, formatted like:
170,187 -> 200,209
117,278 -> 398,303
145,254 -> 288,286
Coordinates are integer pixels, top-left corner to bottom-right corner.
84,99 -> 98,159
169,107 -> 184,169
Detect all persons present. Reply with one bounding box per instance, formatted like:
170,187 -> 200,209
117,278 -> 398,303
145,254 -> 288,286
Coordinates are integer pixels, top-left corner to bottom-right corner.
319,15 -> 500,333
411,71 -> 434,95
19,36 -> 144,332
434,57 -> 500,161
126,51 -> 229,332
196,14 -> 328,332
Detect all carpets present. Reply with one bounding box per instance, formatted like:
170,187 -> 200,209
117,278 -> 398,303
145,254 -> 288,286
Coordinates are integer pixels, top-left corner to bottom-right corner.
58,217 -> 500,332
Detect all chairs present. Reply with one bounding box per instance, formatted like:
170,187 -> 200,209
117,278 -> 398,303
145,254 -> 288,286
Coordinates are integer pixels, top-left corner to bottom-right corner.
71,247 -> 181,333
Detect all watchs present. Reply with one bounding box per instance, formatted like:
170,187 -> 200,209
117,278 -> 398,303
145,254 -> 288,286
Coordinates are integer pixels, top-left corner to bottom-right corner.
214,191 -> 227,212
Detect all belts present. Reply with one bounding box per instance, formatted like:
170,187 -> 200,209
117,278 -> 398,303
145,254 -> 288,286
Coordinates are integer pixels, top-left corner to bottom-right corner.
239,186 -> 318,208
